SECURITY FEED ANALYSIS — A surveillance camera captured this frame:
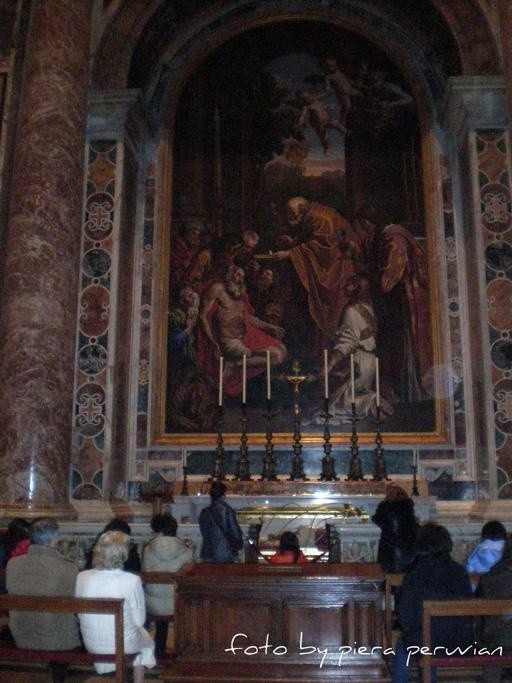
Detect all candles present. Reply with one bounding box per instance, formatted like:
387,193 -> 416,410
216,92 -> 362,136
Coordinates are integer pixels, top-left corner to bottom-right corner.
324,349 -> 328,399
267,351 -> 270,400
242,354 -> 246,403
376,357 -> 379,407
219,357 -> 223,406
351,354 -> 354,403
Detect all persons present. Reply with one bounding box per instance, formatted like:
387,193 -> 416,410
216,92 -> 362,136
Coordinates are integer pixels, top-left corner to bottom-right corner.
388,523 -> 471,683
267,531 -> 309,565
465,521 -> 507,594
2,479 -> 247,683
163,54 -> 434,431
471,536 -> 512,683
368,482 -> 418,625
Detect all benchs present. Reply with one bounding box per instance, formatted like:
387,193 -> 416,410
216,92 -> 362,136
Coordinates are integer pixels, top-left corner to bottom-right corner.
0,571 -> 174,683
385,573 -> 512,683
